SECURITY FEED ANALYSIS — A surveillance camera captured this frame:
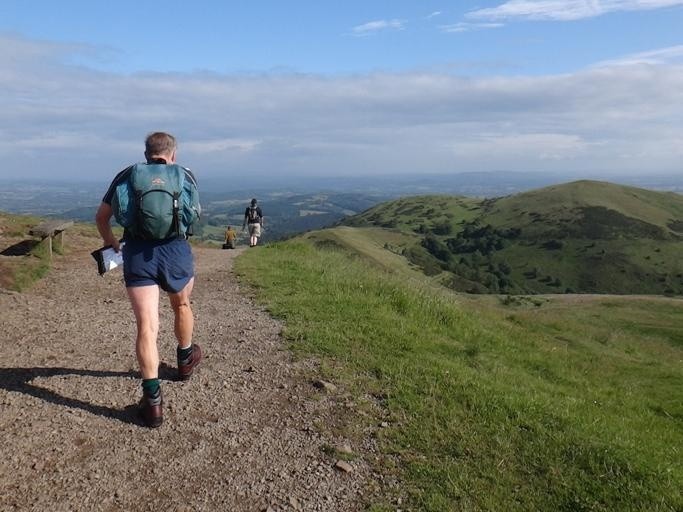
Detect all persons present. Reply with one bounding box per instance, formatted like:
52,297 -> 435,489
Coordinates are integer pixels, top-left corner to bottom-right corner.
240,199 -> 264,246
93,131 -> 202,429
223,226 -> 236,249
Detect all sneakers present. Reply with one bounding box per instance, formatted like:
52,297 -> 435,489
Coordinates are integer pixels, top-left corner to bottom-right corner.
137,386 -> 164,428
177,343 -> 202,379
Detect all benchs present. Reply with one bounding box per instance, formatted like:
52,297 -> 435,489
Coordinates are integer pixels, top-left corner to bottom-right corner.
29,220 -> 74,260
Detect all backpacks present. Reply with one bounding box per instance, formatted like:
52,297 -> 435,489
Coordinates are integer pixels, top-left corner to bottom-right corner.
248,206 -> 259,220
110,162 -> 202,244
